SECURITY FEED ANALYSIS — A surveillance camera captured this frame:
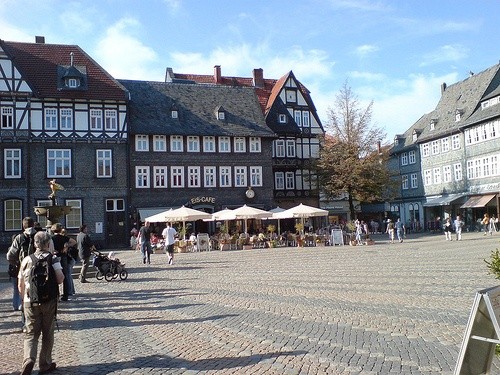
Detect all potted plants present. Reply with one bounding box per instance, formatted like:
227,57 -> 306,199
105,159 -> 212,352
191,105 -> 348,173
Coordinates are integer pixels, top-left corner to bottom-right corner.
240,236 -> 254,251
348,223 -> 358,246
219,224 -> 231,252
267,224 -> 277,248
315,235 -> 326,246
177,225 -> 189,253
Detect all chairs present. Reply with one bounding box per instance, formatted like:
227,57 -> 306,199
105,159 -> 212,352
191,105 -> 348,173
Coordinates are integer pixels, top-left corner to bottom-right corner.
283,232 -> 315,247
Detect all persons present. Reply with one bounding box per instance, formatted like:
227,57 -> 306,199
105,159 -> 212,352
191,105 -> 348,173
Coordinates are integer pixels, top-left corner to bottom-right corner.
138,221 -> 152,264
6,217 -> 115,332
17,230 -> 65,375
476,212 -> 500,235
130,222 -> 159,255
354,217 -> 419,245
48,179 -> 62,200
173,228 -> 201,246
161,222 -> 178,265
209,230 -> 277,249
279,221 -> 345,247
433,213 -> 474,241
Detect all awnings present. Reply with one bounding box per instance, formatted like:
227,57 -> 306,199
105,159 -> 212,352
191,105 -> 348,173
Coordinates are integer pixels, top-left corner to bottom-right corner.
460,194 -> 496,207
423,195 -> 464,206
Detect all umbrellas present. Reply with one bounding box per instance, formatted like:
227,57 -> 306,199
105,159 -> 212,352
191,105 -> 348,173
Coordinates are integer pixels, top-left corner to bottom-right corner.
203,206 -> 237,233
232,203 -> 272,235
165,204 -> 213,240
262,205 -> 287,236
144,208 -> 178,228
283,202 -> 329,231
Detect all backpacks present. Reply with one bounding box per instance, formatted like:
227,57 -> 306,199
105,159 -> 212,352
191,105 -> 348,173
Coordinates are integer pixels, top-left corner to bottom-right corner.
18,233 -> 36,262
28,252 -> 60,304
8,264 -> 19,277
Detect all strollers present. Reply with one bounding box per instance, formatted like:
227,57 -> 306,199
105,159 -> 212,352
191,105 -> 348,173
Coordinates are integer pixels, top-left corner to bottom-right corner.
90,248 -> 128,282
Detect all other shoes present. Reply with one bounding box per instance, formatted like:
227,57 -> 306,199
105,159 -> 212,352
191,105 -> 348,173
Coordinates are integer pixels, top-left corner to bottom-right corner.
20,358 -> 36,375
143,257 -> 146,264
168,256 -> 173,264
147,260 -> 150,264
17,305 -> 23,311
38,362 -> 56,373
